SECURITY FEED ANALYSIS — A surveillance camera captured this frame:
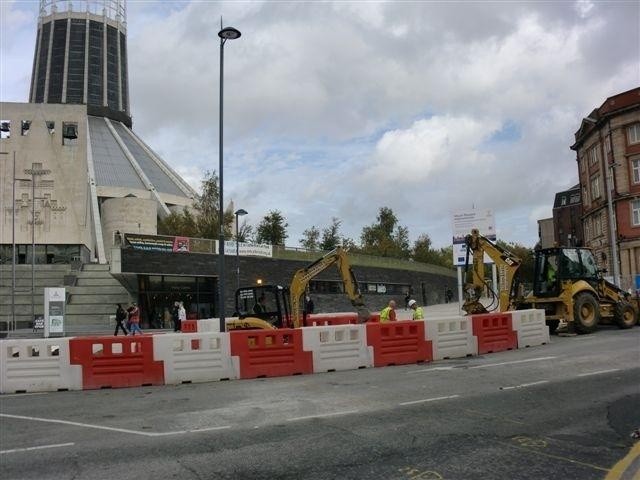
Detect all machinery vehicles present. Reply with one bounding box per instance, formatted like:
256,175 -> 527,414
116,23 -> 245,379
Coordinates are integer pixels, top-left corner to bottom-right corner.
461,230 -> 639,333
224,247 -> 372,331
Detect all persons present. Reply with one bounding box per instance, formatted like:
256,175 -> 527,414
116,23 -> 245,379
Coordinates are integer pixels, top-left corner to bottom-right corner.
113,304 -> 128,336
170,301 -> 179,331
177,300 -> 186,333
547,261 -> 558,281
405,292 -> 411,311
408,299 -> 425,320
126,302 -> 135,336
129,302 -> 145,336
447,288 -> 454,303
380,300 -> 398,321
253,295 -> 268,313
305,296 -> 314,313
126,302 -> 137,336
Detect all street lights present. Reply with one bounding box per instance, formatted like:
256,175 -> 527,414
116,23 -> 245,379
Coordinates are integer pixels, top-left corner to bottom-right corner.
218,13 -> 240,333
233,205 -> 248,289
12,152 -> 33,335
